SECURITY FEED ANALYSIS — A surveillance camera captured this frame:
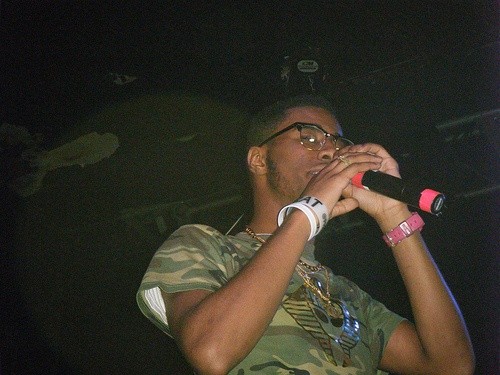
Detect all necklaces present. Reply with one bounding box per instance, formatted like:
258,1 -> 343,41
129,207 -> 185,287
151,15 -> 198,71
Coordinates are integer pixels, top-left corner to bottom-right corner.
242,222 -> 339,316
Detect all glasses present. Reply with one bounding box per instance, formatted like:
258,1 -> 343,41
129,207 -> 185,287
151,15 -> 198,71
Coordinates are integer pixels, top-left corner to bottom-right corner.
260,122 -> 355,153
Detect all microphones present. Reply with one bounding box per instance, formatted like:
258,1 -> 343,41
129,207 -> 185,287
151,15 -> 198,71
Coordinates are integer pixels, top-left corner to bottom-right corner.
348,156 -> 446,214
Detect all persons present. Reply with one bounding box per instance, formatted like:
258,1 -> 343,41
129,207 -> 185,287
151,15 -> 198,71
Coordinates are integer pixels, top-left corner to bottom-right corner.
135,93 -> 476,375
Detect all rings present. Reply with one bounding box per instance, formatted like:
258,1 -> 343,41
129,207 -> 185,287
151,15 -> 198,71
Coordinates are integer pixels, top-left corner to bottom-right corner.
337,154 -> 350,167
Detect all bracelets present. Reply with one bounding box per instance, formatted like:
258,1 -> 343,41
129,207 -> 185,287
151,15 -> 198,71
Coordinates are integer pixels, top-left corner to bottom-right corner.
382,212 -> 426,248
277,191 -> 329,243
